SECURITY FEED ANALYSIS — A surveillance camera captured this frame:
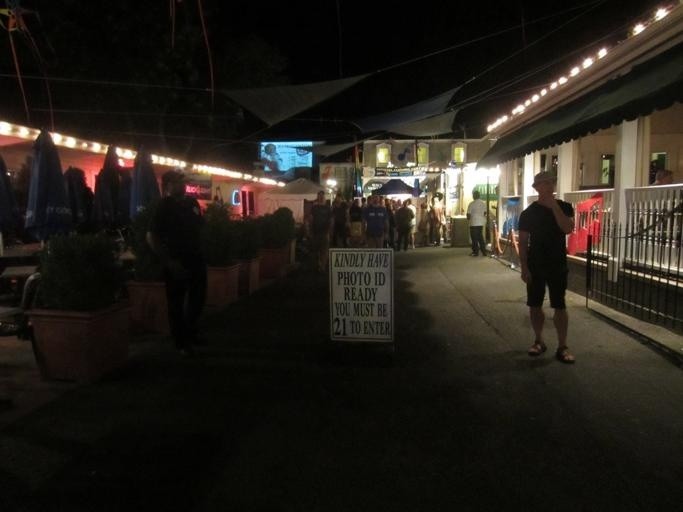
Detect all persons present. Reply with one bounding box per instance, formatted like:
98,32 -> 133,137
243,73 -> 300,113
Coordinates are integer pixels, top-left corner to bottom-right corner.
262,144 -> 282,171
306,187 -> 488,274
147,169 -> 211,357
517,171 -> 575,363
651,168 -> 675,185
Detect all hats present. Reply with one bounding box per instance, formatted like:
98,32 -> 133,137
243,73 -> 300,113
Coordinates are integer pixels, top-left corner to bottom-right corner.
533,172 -> 557,187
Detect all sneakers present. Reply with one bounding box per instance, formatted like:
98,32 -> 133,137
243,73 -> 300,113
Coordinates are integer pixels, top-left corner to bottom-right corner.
469,252 -> 478,256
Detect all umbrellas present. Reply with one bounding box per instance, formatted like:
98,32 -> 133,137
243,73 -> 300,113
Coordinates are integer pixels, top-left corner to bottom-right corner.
0,125 -> 163,249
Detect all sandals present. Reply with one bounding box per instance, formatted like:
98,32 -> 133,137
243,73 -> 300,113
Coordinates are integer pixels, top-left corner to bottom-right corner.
528,340 -> 547,356
556,345 -> 576,364
